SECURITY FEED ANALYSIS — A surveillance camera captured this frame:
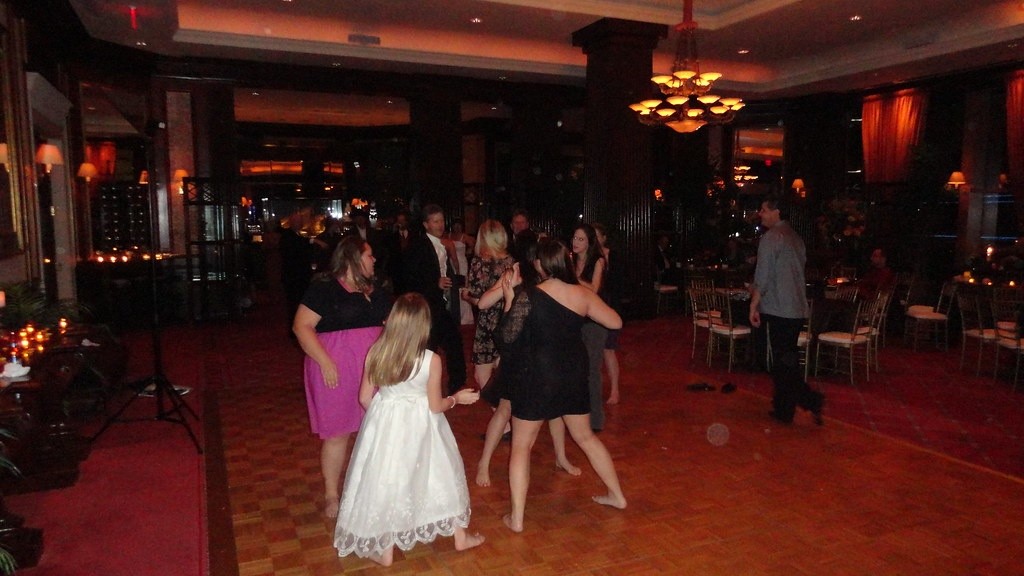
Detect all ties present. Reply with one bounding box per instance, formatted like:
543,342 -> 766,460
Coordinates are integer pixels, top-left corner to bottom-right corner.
402,232 -> 405,239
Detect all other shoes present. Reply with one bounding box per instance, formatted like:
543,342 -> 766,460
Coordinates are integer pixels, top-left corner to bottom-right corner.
767,409 -> 794,427
721,383 -> 737,394
812,391 -> 826,426
480,430 -> 512,441
686,382 -> 716,392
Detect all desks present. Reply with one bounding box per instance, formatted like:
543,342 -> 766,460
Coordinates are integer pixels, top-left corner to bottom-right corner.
954,274 -> 1024,343
718,278 -> 851,370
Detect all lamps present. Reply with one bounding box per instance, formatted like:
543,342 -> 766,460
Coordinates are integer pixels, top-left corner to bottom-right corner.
0,142 -> 9,172
791,179 -> 804,193
76,162 -> 96,183
35,144 -> 64,176
138,172 -> 149,184
628,0 -> 746,134
948,171 -> 965,193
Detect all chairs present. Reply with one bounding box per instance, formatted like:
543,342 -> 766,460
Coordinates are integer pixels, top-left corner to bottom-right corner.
653,262 -> 1024,386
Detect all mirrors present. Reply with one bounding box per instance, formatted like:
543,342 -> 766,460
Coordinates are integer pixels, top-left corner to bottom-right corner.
0,0 -> 24,262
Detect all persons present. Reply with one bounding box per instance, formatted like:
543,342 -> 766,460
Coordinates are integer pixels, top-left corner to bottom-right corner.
385,203 -> 625,487
495,235 -> 627,531
717,233 -> 757,268
652,229 -> 687,306
748,192 -> 826,425
809,246 -> 904,377
359,293 -> 486,567
278,201 -> 376,340
953,235 -> 1024,342
713,273 -> 767,366
292,239 -> 399,519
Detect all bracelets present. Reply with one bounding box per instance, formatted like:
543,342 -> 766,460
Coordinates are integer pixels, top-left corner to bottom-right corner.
446,396 -> 456,409
